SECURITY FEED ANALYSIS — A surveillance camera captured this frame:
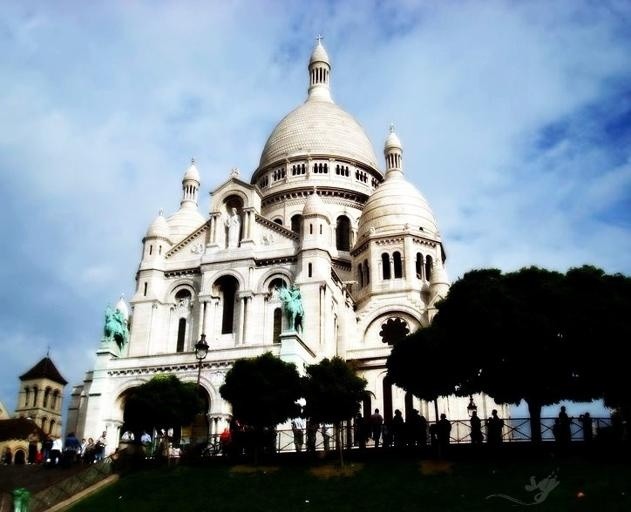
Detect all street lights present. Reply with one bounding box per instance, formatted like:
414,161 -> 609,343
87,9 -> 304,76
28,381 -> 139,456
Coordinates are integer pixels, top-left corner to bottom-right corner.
194,333 -> 209,383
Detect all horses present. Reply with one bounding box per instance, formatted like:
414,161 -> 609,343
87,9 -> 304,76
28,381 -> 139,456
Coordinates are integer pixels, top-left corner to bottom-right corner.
103,304 -> 129,353
278,283 -> 305,335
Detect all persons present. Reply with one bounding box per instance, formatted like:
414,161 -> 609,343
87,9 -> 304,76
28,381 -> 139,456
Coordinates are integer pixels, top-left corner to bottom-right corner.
292,284 -> 301,302
552,406 -> 573,437
27,428 -> 107,463
220,424 -> 276,456
470,410 -> 504,444
115,308 -> 125,326
578,412 -> 593,441
292,422 -> 302,452
353,409 -> 452,448
122,428 -> 182,463
